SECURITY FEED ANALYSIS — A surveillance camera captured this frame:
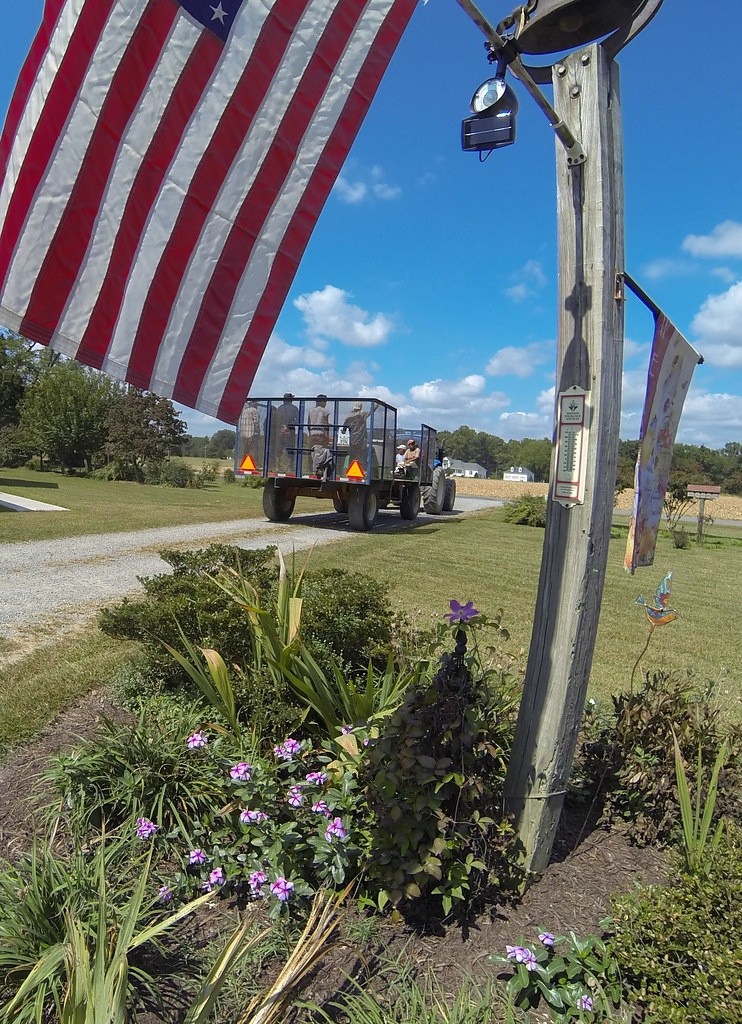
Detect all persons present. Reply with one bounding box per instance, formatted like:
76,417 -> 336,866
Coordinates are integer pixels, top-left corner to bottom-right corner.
390,438 -> 422,481
306,393 -> 336,477
262,391 -> 301,475
237,399 -> 262,469
339,401 -> 382,479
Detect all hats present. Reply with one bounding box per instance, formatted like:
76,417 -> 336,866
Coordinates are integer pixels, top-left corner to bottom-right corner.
352,403 -> 363,410
407,439 -> 415,446
397,444 -> 407,450
283,393 -> 295,398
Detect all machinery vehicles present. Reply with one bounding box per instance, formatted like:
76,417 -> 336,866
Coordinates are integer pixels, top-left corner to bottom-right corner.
234,395 -> 457,531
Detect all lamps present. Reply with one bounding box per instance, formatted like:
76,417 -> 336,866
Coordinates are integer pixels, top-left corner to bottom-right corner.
460,33 -> 521,152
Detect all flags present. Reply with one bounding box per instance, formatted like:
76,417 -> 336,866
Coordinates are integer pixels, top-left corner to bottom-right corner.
0,0 -> 419,428
